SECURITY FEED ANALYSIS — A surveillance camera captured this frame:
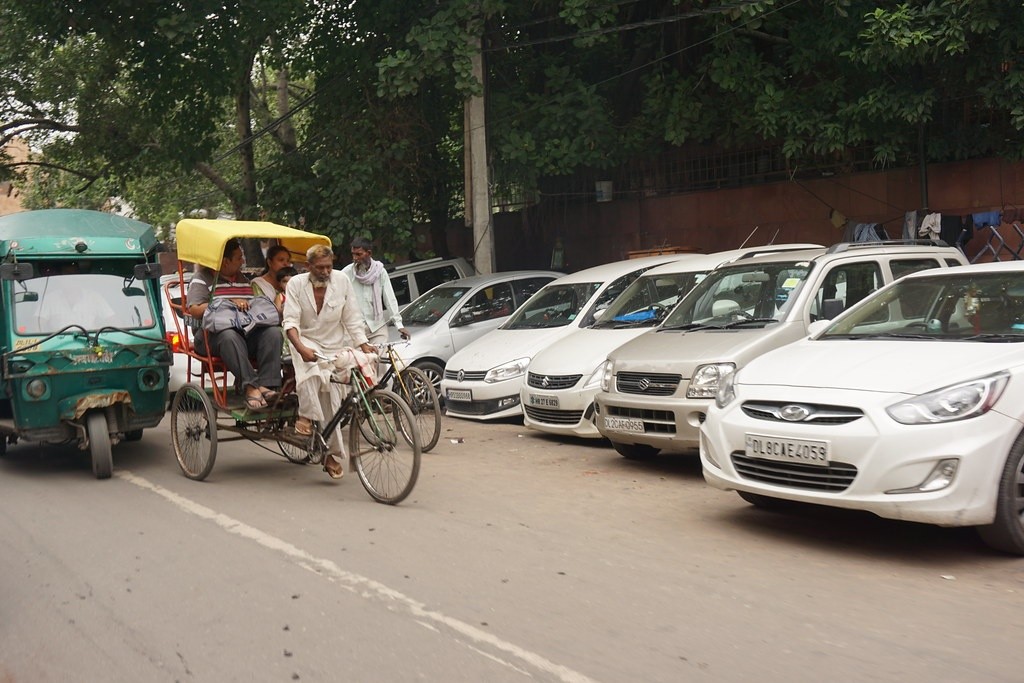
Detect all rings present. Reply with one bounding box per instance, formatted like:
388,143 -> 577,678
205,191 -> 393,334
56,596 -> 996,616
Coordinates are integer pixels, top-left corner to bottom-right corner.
241,302 -> 244,306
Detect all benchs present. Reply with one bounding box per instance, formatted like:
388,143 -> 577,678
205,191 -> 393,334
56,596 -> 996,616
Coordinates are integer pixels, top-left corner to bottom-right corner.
166,279 -> 259,368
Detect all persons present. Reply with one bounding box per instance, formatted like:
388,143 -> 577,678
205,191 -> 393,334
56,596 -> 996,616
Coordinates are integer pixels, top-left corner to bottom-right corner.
340,236 -> 411,379
33,260 -> 119,332
251,245 -> 299,356
281,244 -> 380,480
186,238 -> 283,410
429,288 -> 511,323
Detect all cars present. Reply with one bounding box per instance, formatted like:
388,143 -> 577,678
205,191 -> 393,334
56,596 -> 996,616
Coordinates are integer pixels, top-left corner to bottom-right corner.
439,253 -> 708,420
594,239 -> 980,459
520,243 -> 824,438
355,271 -> 580,411
699,260 -> 1024,552
157,272 -> 284,392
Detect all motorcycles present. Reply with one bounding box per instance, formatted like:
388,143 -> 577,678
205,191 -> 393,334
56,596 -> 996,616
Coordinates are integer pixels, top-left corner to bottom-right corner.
1,209 -> 173,479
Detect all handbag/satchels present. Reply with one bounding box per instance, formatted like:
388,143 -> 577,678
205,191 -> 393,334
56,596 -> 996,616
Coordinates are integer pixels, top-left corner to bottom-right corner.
202,295 -> 280,336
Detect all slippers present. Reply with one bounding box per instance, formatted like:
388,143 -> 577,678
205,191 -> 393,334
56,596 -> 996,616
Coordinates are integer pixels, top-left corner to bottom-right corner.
295,417 -> 312,435
262,390 -> 280,401
378,401 -> 392,415
242,392 -> 268,410
322,459 -> 344,478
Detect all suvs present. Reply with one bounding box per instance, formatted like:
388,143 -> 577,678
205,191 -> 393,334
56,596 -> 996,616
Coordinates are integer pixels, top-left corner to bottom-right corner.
381,255 -> 474,323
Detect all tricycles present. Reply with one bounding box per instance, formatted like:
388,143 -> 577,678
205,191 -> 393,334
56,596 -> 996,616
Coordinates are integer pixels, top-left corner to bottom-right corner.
170,219 -> 421,506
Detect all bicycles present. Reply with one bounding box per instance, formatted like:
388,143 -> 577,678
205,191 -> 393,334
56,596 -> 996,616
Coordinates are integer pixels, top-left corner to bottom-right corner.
329,333 -> 441,453
